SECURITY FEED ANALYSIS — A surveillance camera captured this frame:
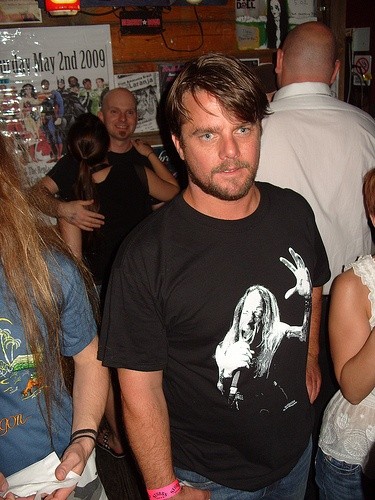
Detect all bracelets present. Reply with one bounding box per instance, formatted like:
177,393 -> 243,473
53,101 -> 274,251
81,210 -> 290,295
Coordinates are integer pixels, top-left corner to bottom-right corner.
147,151 -> 155,157
69,429 -> 97,444
144,478 -> 184,500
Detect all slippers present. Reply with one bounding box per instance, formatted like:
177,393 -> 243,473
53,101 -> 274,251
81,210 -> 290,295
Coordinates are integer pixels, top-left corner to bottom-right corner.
96,424 -> 125,458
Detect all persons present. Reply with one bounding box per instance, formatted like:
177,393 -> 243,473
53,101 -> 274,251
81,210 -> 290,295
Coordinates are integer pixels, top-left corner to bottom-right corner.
252,21 -> 375,466
0,127 -> 111,500
312,169 -> 375,500
0,76 -> 106,162
56,111 -> 184,460
97,52 -> 332,500
28,87 -> 169,231
